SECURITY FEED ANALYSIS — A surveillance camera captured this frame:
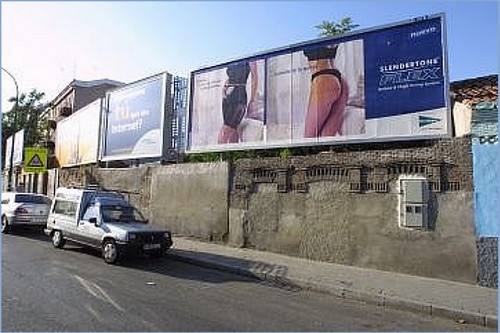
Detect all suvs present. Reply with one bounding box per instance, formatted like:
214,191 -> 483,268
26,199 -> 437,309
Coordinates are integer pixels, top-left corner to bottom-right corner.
47,186 -> 173,266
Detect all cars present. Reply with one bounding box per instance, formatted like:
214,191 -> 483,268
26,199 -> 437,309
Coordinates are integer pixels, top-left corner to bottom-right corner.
2,191 -> 50,236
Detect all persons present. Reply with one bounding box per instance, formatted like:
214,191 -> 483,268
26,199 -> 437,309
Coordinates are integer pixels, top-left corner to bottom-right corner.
301,43 -> 350,138
216,61 -> 259,144
